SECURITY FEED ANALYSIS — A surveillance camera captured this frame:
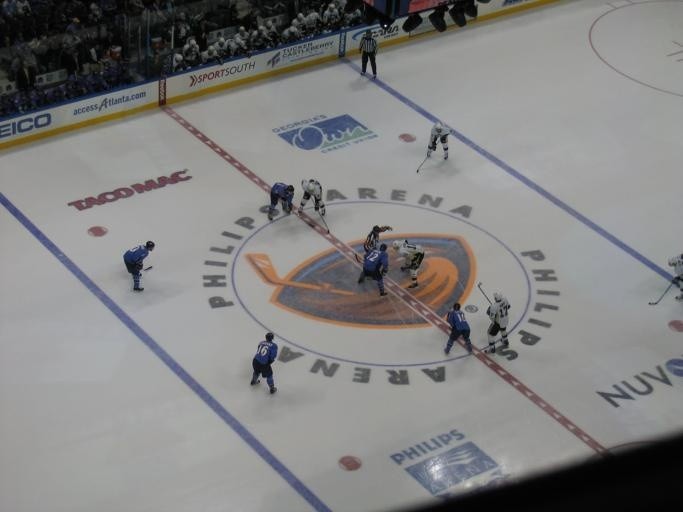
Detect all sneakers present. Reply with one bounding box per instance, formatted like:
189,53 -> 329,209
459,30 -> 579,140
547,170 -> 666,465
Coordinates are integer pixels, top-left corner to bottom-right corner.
358,266 -> 418,297
132,273 -> 144,291
268,207 -> 327,220
444,339 -> 509,353
426,150 -> 449,160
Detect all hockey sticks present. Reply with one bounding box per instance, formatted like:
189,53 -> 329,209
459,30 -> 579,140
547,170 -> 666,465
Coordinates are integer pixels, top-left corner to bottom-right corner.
354,254 -> 386,277
476,281 -> 493,306
142,265 -> 151,271
308,195 -> 329,234
291,210 -> 316,228
648,283 -> 675,305
416,156 -> 428,177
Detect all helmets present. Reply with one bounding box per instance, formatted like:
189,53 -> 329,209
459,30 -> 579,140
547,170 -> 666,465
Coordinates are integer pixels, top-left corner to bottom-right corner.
265,332 -> 274,341
288,183 -> 315,196
435,123 -> 442,133
454,291 -> 502,311
373,224 -> 401,252
146,240 -> 154,252
174,4 -> 335,62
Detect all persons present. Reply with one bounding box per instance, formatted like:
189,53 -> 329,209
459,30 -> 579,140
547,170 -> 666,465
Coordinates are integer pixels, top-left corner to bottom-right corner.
426,121 -> 450,160
667,253 -> 683,300
483,291 -> 511,354
364,225 -> 393,252
357,243 -> 389,299
268,182 -> 295,221
392,238 -> 425,289
250,333 -> 278,394
123,241 -> 155,291
297,178 -> 326,219
442,302 -> 472,356
